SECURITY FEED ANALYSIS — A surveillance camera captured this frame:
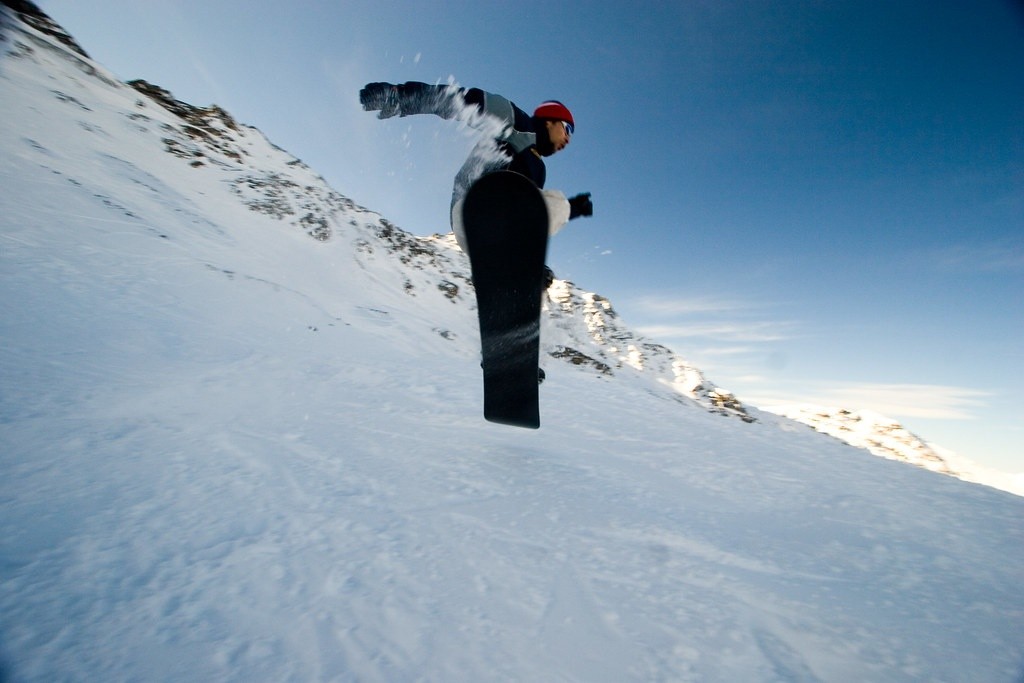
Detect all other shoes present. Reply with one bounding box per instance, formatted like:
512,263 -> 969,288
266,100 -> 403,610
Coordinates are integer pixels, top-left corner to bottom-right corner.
542,265 -> 553,291
480,360 -> 546,384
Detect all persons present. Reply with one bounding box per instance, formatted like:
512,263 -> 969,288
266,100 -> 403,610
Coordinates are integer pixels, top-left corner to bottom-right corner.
359,80 -> 594,384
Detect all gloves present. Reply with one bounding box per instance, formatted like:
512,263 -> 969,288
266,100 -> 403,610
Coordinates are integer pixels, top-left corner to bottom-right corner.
567,192 -> 593,219
360,82 -> 400,120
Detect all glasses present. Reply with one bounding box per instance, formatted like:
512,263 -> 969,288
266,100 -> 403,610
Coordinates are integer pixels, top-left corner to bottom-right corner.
561,121 -> 573,135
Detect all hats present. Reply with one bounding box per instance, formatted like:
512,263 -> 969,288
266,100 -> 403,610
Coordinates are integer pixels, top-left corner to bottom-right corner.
534,99 -> 575,126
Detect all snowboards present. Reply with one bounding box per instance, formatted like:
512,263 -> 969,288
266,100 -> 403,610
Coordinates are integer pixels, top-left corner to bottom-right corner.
461,171 -> 549,432
